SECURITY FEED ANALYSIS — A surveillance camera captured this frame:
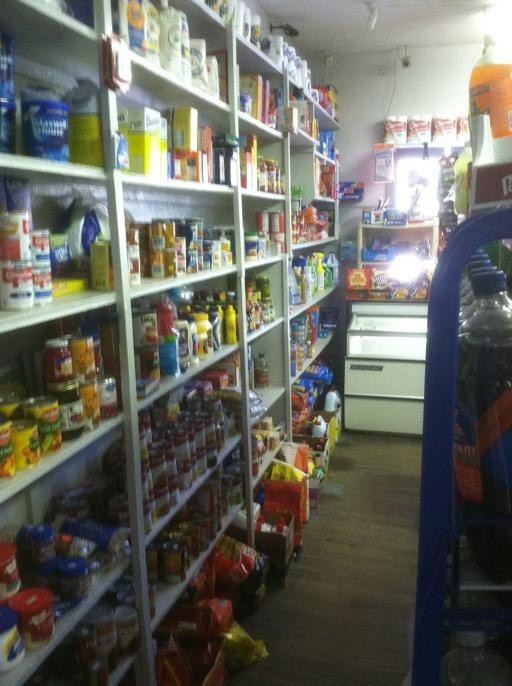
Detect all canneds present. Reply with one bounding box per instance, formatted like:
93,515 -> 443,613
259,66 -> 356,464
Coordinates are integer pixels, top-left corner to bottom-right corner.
255,211 -> 286,255
132,308 -> 160,383
20,98 -> 72,160
27,576 -> 156,686
108,398 -> 225,536
258,157 -> 286,197
0,329 -> 118,480
0,33 -> 15,153
146,464 -> 230,619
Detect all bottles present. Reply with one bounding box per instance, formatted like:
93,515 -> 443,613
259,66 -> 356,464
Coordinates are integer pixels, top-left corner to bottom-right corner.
255,353 -> 270,386
442,247 -> 512,589
259,155 -> 283,195
180,294 -> 242,368
308,251 -> 333,291
46,336 -> 89,440
